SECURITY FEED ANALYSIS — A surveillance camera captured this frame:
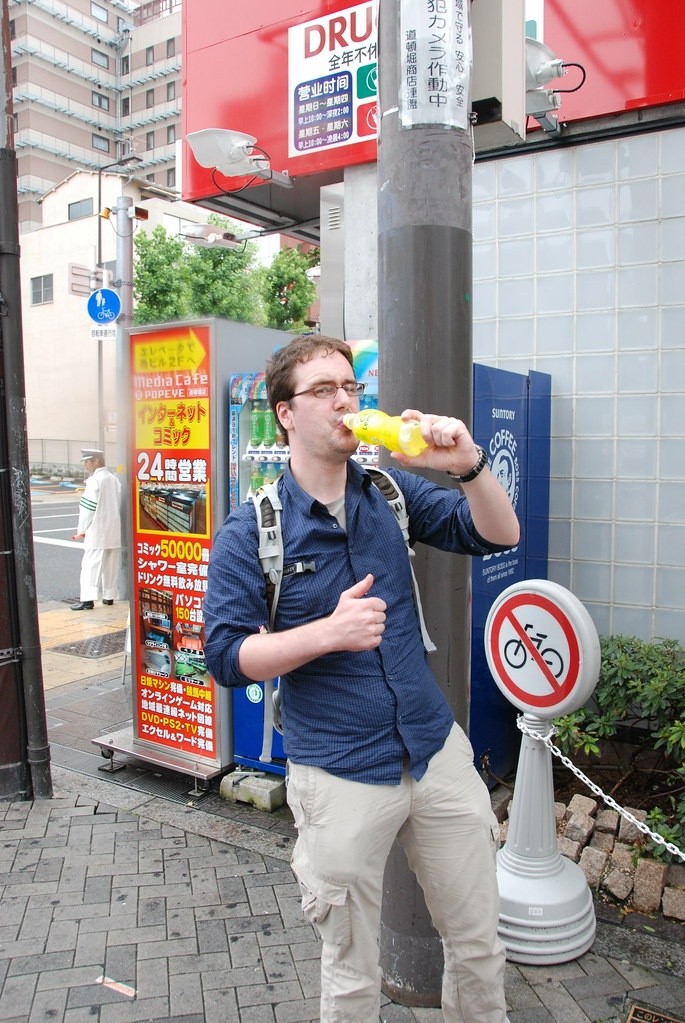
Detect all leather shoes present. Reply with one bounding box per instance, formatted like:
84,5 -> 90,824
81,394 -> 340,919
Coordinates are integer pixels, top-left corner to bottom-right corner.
70,601 -> 94,611
103,599 -> 113,605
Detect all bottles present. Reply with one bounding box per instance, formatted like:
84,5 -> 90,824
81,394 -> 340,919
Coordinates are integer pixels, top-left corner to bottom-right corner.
250,462 -> 263,496
343,409 -> 428,458
250,402 -> 264,447
264,463 -> 277,486
263,401 -> 276,447
276,423 -> 286,449
277,464 -> 286,478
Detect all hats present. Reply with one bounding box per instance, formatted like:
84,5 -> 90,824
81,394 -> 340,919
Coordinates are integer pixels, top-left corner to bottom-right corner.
79,449 -> 104,462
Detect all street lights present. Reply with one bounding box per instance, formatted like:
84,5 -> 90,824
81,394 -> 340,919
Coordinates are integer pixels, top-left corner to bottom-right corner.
97,156 -> 143,461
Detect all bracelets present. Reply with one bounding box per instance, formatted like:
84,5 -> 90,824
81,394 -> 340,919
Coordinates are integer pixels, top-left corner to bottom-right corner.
447,444 -> 488,483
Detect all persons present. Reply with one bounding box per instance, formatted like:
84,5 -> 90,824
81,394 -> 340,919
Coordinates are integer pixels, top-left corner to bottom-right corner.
70,449 -> 121,610
204,337 -> 520,1022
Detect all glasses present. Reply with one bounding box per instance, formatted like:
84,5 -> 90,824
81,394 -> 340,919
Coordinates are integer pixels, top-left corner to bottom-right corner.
287,383 -> 365,399
